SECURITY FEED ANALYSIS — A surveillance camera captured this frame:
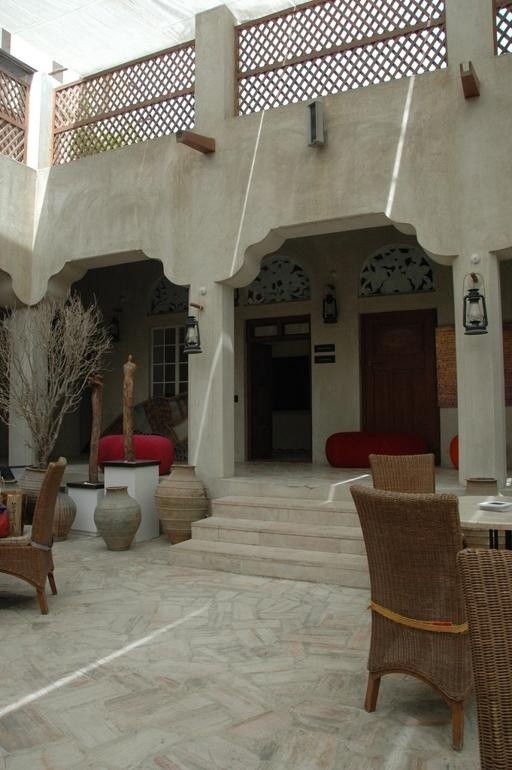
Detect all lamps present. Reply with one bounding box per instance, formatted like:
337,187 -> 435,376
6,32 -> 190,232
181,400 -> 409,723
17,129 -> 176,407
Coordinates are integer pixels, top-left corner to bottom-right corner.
462,272 -> 488,335
322,284 -> 338,324
182,303 -> 204,354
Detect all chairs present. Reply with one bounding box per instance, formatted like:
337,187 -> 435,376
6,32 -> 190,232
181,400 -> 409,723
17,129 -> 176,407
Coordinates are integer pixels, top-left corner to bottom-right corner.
349,484 -> 475,752
457,548 -> 512,770
0,456 -> 67,615
369,453 -> 435,494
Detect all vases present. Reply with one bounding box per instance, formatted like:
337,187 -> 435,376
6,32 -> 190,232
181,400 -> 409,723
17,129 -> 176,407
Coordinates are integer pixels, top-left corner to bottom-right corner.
0,465 -> 208,552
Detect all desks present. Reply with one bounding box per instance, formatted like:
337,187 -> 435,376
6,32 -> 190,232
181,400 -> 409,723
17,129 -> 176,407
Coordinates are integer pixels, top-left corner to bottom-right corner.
457,495 -> 512,550
